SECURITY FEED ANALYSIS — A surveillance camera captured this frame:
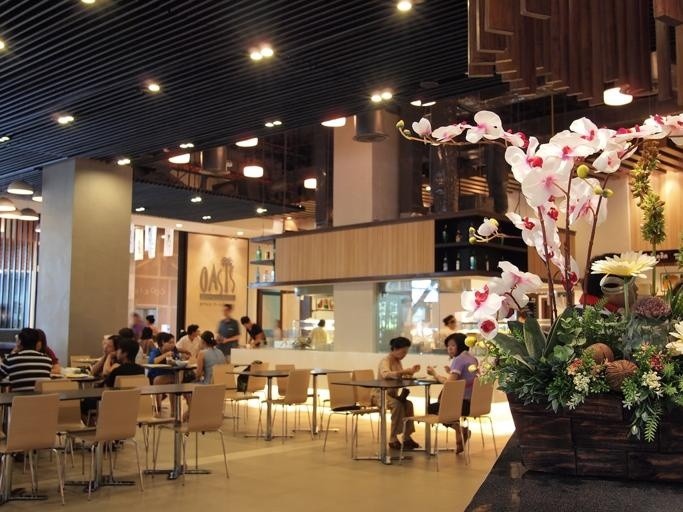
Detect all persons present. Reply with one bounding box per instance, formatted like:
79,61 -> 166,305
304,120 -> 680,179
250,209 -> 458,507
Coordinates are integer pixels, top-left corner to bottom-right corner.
240,316 -> 266,346
310,320 -> 328,351
131,312 -> 146,342
440,315 -> 458,339
216,304 -> 241,363
368,337 -> 422,450
575,252 -> 640,317
79,325 -> 271,453
145,314 -> 161,336
426,333 -> 479,457
0,328 -> 52,462
11,328 -> 63,378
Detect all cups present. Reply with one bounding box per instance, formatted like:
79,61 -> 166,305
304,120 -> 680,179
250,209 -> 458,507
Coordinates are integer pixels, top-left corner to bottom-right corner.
179,352 -> 185,361
165,354 -> 172,364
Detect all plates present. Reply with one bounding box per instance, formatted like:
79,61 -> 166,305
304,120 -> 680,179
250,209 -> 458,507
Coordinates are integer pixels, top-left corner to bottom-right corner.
67,373 -> 86,378
418,378 -> 435,381
50,373 -> 63,377
142,363 -> 170,368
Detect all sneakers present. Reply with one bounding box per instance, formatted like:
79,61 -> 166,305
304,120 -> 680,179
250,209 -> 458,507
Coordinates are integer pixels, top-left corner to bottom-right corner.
13,453 -> 24,464
389,439 -> 419,450
455,427 -> 470,455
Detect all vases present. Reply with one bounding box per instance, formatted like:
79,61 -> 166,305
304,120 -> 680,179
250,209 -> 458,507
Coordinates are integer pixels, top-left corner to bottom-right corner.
503,390 -> 683,490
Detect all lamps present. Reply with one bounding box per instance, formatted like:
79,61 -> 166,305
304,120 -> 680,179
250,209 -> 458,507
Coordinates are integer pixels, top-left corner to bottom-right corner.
0,179 -> 42,234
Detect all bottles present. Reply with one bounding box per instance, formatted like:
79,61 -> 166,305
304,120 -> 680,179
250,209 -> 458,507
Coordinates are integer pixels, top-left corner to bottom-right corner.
316,295 -> 333,309
442,224 -> 504,273
255,245 -> 275,283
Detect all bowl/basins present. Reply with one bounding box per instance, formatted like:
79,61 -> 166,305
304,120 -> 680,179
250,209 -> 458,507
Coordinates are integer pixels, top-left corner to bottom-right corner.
175,361 -> 187,366
65,368 -> 80,373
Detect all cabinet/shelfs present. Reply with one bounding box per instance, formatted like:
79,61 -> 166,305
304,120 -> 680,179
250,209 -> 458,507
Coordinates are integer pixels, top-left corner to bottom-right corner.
248,237 -> 275,288
435,209 -> 528,276
